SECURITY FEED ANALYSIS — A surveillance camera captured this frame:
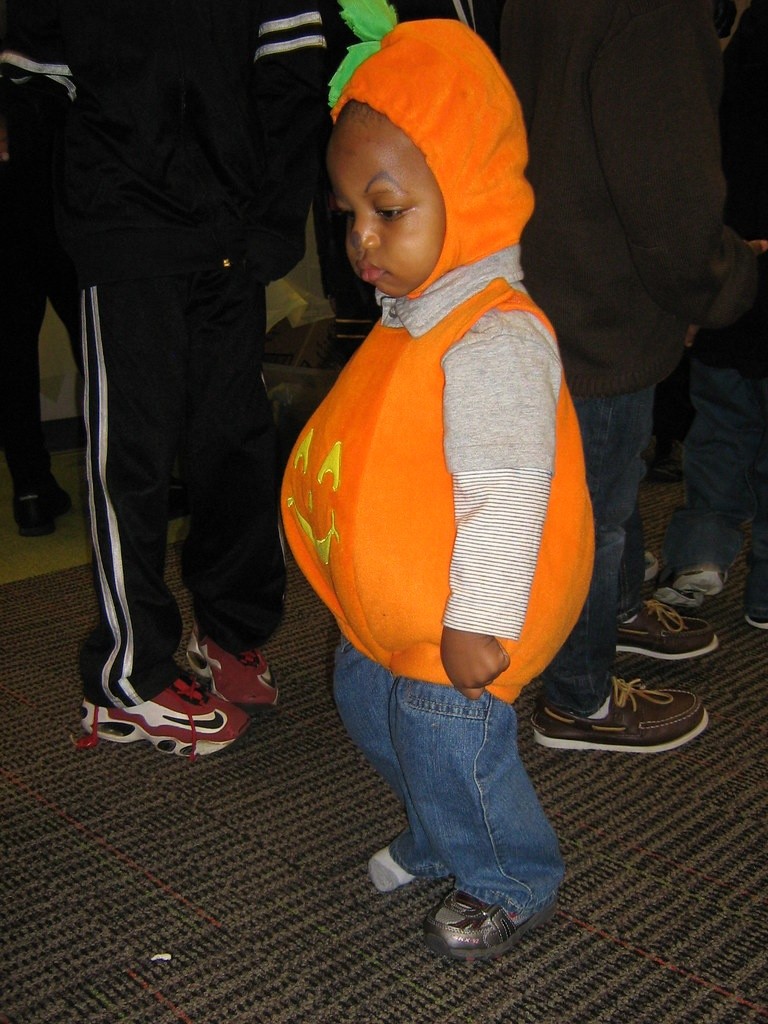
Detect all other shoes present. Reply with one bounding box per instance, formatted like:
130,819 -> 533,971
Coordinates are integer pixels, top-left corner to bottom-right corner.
744,613 -> 768,630
13,481 -> 72,537
651,562 -> 724,609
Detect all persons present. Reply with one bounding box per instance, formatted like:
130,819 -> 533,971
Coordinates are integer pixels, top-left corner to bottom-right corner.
0,0 -> 768,754
281,0 -> 596,958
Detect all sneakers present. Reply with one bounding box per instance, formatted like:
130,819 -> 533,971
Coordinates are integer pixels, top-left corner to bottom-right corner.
614,599 -> 717,660
531,675 -> 709,753
76,671 -> 253,762
423,886 -> 559,960
185,614 -> 278,705
